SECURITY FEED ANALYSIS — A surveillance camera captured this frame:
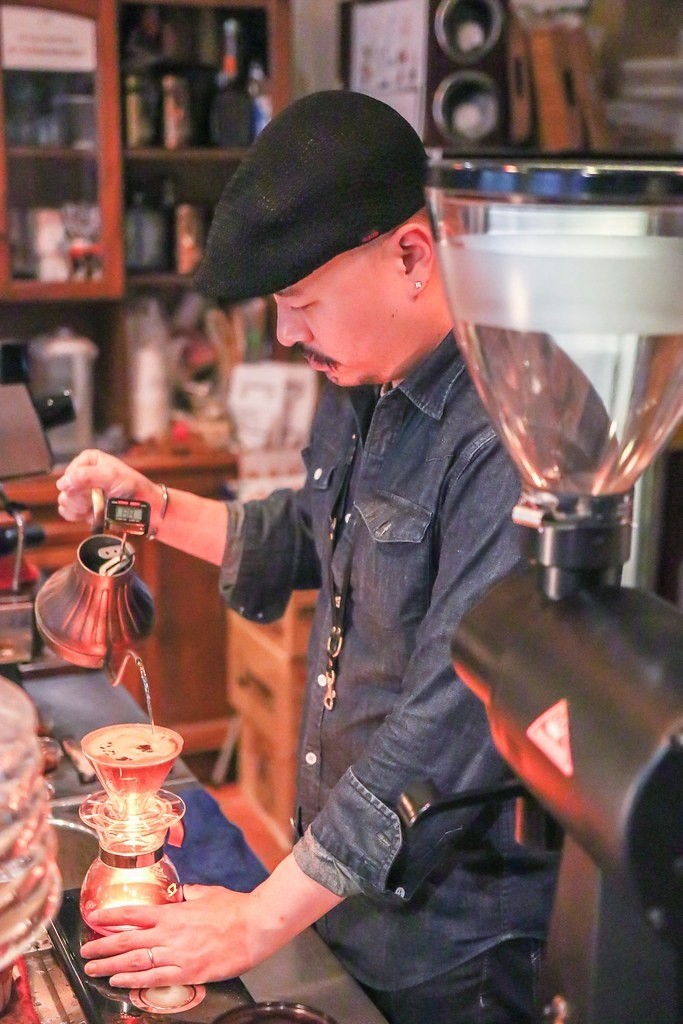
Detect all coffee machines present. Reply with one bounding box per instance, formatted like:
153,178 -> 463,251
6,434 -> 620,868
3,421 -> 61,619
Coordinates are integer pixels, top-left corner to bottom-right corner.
422,154 -> 683,1020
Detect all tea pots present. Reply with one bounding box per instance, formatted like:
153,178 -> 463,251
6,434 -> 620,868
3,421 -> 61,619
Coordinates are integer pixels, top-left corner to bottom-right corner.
35,486 -> 154,687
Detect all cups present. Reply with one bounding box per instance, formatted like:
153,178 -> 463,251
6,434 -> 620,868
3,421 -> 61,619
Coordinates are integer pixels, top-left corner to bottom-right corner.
79,724 -> 186,832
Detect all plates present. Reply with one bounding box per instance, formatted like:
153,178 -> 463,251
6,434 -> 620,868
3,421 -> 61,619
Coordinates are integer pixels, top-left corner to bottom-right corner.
211,1002 -> 338,1024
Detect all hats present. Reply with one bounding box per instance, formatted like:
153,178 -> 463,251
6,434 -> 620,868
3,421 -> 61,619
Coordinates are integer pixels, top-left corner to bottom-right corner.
193,90 -> 427,309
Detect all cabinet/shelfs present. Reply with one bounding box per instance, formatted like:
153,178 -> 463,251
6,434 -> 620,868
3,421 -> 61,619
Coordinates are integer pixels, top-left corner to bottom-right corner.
0,1 -> 318,751
226,589 -> 319,849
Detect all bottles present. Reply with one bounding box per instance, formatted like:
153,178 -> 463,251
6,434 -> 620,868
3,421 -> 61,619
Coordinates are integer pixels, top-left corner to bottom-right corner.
204,19 -> 254,149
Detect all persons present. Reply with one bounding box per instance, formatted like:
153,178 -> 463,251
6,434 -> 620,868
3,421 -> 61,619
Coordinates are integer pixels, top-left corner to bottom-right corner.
53,89 -> 632,1024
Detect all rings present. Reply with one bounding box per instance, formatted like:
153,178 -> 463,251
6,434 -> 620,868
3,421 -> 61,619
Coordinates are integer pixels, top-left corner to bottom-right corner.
147,947 -> 156,969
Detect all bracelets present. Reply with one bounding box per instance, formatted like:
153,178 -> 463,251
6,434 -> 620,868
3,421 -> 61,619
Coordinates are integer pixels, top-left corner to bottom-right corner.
143,483 -> 169,541
